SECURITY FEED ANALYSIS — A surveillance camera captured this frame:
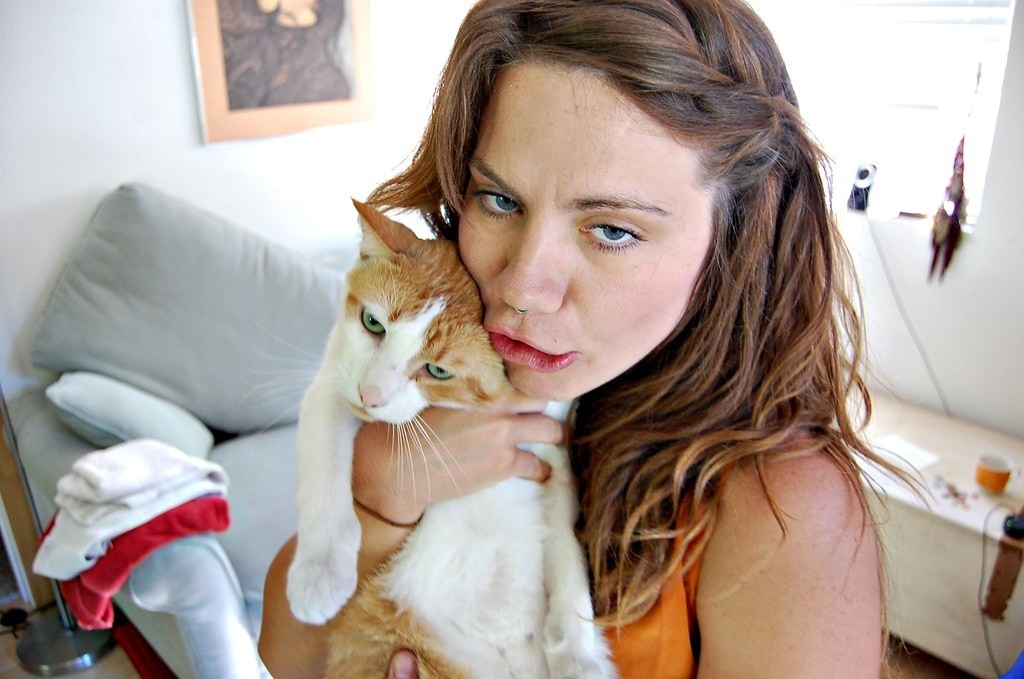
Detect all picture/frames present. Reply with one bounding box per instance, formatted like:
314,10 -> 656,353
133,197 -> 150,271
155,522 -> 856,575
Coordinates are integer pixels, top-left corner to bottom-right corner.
187,1 -> 378,146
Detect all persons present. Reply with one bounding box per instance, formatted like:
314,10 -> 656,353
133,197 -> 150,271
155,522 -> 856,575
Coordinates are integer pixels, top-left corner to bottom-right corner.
256,0 -> 935,679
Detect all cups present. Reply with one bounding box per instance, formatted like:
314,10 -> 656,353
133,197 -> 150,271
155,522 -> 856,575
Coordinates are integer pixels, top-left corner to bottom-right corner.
972,452 -> 1014,496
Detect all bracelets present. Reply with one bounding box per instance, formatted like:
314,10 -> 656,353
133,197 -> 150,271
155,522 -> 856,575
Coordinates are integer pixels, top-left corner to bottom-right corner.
353,497 -> 424,528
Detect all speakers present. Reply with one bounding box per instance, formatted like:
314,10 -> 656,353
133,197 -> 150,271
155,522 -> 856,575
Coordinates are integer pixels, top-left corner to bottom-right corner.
847,163 -> 876,212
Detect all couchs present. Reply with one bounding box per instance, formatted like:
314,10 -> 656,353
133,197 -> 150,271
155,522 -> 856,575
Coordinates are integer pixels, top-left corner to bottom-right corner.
6,379 -> 301,678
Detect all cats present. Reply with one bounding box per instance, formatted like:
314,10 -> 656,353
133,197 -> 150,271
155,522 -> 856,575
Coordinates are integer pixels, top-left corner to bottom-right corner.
285,198 -> 622,679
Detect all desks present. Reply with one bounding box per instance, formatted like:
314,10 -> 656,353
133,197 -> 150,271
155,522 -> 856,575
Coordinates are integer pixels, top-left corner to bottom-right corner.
838,388 -> 1024,679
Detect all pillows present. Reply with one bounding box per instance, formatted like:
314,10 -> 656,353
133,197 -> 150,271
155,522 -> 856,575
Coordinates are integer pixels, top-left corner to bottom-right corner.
44,372 -> 214,465
29,179 -> 353,433
